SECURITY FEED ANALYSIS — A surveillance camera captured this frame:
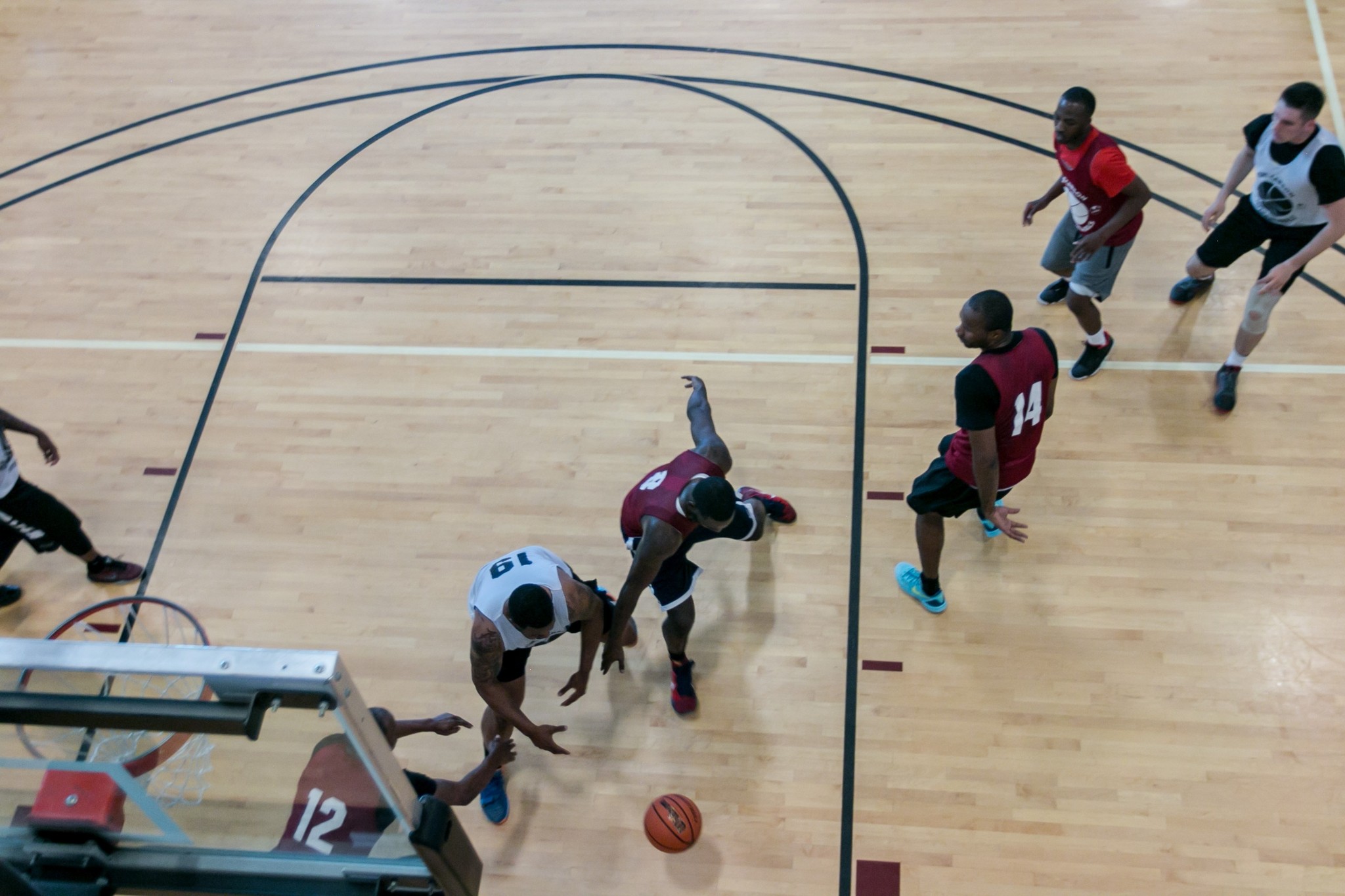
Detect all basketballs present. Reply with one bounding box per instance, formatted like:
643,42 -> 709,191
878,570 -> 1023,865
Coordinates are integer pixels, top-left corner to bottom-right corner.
644,794 -> 702,853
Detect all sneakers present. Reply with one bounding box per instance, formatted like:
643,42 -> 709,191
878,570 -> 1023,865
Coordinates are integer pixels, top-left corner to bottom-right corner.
975,498 -> 1005,537
86,554 -> 144,583
479,768 -> 509,825
1037,277 -> 1070,306
737,486 -> 797,524
0,584 -> 22,608
895,561 -> 947,613
1170,274 -> 1216,304
667,658 -> 698,715
1069,330 -> 1115,380
596,585 -> 615,602
1213,360 -> 1242,412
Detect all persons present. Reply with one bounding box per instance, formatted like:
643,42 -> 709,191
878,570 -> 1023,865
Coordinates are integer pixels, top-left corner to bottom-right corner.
1021,87 -> 1154,381
270,708 -> 517,859
601,375 -> 798,716
467,545 -> 638,826
0,407 -> 146,608
1168,83 -> 1345,413
898,290 -> 1057,614
